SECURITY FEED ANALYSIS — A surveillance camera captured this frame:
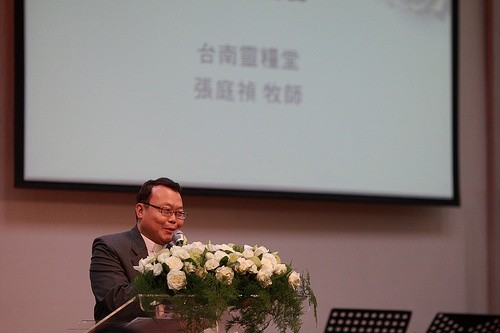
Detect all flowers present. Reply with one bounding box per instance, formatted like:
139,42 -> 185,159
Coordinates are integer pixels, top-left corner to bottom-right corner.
131,236 -> 318,333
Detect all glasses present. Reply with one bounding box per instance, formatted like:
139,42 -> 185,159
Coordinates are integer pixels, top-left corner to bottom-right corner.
138,201 -> 189,220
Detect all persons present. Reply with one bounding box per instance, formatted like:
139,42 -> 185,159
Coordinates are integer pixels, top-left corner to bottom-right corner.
89,177 -> 188,323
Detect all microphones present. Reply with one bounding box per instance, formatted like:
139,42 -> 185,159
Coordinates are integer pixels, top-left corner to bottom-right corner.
172,230 -> 184,247
